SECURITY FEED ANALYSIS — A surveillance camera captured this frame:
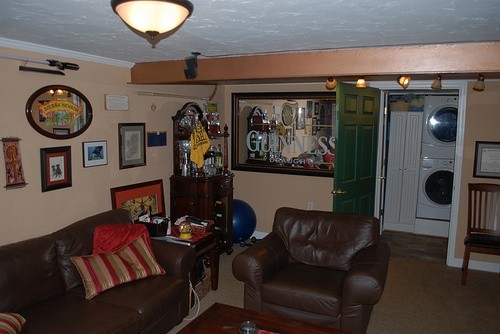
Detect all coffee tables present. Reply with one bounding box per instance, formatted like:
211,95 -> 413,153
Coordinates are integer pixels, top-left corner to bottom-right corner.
175,302 -> 359,334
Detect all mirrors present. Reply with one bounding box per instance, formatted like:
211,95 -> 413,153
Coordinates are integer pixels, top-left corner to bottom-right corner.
25,85 -> 93,140
231,92 -> 336,178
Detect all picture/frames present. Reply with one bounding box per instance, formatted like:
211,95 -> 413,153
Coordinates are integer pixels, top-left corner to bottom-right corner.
296,101 -> 321,136
118,122 -> 146,170
40,145 -> 73,193
110,179 -> 166,224
82,140 -> 108,168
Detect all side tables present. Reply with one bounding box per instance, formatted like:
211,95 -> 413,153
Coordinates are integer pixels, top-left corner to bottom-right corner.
162,220 -> 222,292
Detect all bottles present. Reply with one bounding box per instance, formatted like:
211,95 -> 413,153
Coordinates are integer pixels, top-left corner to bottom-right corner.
248,105 -> 278,160
177,113 -> 224,179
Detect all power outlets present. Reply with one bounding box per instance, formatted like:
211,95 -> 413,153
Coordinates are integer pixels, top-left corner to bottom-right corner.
307,202 -> 313,210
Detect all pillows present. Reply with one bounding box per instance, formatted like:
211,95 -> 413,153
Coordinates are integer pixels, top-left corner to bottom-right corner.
69,233 -> 166,301
0,313 -> 26,334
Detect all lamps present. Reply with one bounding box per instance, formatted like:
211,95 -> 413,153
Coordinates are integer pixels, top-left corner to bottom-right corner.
472,75 -> 485,90
111,0 -> 193,40
396,75 -> 411,89
355,77 -> 367,88
325,77 -> 337,91
431,74 -> 442,90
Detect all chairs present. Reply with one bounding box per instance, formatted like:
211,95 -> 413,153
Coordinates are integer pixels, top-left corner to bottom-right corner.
461,183 -> 500,284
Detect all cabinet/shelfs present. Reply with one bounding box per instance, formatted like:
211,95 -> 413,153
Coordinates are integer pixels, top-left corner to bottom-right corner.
169,102 -> 235,256
245,106 -> 288,164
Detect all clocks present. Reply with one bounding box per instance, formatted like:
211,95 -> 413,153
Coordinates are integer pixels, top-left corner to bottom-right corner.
282,105 -> 293,126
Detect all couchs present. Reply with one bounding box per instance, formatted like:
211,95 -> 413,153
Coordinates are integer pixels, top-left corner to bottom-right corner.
232,207 -> 391,334
0,209 -> 206,334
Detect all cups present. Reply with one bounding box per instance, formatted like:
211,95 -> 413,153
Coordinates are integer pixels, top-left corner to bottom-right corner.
238,320 -> 258,334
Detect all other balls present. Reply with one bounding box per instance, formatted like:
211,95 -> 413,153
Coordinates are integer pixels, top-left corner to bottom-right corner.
231,199 -> 257,244
